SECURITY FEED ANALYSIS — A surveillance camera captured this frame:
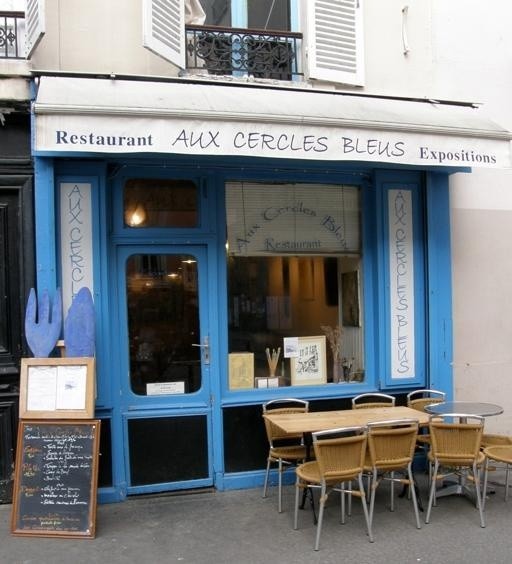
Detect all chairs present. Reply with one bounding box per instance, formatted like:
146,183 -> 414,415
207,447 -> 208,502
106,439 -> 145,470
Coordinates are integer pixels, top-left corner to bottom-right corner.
294,425 -> 375,551
479,433 -> 512,448
407,389 -> 446,409
425,412 -> 488,528
262,397 -> 316,513
364,419 -> 423,534
483,445 -> 512,513
351,392 -> 395,409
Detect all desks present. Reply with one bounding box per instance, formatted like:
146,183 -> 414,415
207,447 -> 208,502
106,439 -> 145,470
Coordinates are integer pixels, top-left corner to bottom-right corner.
425,401 -> 504,508
263,406 -> 444,524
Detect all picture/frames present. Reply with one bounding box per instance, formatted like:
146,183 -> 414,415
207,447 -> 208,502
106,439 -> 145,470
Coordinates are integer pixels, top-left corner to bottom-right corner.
290,335 -> 328,386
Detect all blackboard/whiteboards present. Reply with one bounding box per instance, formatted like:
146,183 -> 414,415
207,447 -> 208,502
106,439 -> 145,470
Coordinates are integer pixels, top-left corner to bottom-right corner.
11,420 -> 102,540
